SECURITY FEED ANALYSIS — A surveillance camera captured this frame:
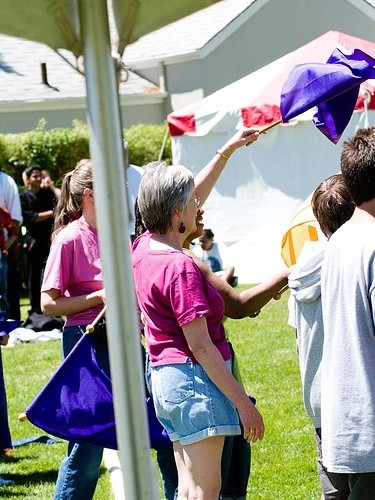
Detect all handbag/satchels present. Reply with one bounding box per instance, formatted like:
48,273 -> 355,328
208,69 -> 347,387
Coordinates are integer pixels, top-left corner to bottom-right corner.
19,299 -> 174,451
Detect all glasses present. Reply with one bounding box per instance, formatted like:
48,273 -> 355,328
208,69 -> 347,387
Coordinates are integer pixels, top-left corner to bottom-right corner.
188,194 -> 201,207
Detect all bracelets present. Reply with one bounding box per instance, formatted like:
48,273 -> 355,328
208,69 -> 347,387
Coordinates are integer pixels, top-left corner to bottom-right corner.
216,151 -> 230,161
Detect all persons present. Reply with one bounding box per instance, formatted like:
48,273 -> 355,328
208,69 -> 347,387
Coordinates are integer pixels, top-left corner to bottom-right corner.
0,126 -> 375,500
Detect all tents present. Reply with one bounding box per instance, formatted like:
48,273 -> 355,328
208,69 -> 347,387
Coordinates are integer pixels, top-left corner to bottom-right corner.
165,27 -> 375,284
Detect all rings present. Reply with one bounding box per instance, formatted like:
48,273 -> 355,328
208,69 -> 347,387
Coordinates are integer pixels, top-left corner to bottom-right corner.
249,428 -> 254,435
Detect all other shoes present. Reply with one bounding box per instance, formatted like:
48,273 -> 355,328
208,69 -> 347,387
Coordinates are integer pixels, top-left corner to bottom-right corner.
0,475 -> 14,486
